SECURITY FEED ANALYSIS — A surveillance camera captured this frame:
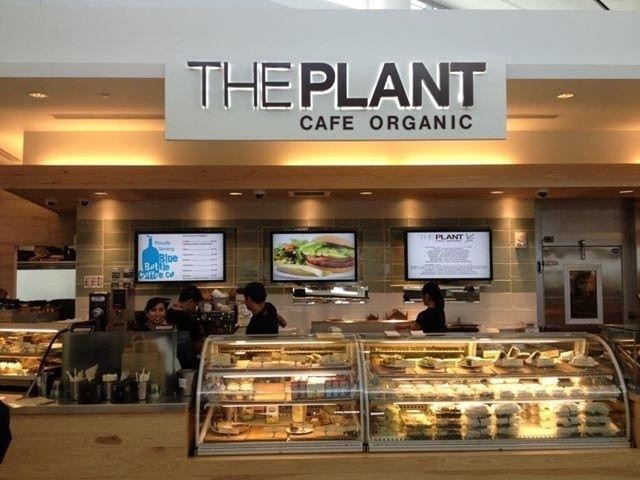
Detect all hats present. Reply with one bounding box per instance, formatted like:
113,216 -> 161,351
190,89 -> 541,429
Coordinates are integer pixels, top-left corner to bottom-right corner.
236,282 -> 264,294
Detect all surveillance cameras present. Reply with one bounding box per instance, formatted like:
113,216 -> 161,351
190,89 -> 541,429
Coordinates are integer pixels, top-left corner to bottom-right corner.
253,189 -> 265,199
46,199 -> 58,207
80,200 -> 91,207
536,188 -> 550,199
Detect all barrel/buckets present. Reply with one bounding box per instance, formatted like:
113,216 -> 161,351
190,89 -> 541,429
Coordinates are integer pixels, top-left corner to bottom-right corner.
177,370 -> 197,397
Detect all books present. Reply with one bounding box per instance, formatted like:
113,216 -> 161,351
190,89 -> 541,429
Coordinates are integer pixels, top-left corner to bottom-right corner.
153,324 -> 177,330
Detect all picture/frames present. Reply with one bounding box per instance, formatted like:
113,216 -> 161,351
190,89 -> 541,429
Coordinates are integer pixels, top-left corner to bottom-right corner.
563,263 -> 604,325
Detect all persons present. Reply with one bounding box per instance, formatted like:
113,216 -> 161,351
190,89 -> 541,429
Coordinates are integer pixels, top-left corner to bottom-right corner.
243,281 -> 288,335
135,297 -> 183,377
166,285 -> 205,369
410,281 -> 447,334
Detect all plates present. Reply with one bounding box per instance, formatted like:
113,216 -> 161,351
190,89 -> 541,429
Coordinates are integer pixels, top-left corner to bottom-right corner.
273,264 -> 355,279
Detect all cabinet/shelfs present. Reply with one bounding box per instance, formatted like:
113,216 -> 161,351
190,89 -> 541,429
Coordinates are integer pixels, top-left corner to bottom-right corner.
357,332 -> 631,453
0,318 -> 73,387
194,332 -> 364,455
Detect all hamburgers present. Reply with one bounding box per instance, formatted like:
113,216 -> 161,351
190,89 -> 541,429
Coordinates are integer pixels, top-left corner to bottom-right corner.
296,235 -> 355,273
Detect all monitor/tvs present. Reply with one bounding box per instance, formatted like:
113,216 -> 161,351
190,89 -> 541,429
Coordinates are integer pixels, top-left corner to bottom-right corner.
134,230 -> 227,284
403,229 -> 493,281
268,230 -> 359,283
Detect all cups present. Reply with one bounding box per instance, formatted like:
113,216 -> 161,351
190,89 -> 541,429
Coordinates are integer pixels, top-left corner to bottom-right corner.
111,268 -> 131,292
70,379 -> 151,401
